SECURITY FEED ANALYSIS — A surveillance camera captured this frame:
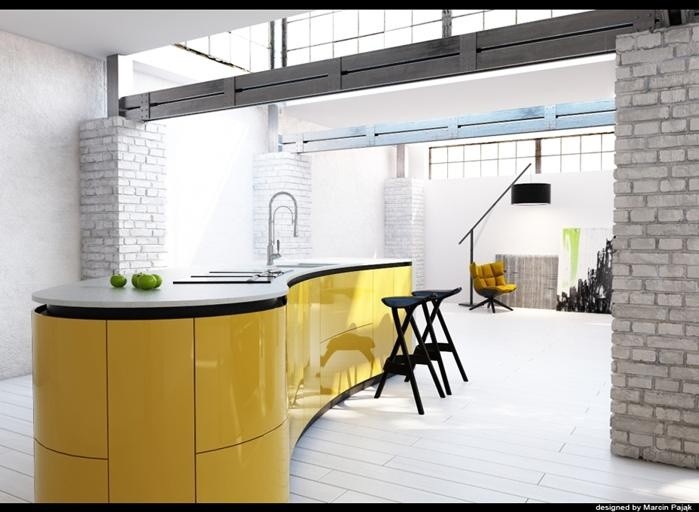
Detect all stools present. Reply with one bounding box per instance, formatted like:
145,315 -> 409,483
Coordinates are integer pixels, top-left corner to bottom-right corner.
405,287 -> 468,396
374,294 -> 445,415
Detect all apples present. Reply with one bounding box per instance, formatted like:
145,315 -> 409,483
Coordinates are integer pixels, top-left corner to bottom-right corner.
132,273 -> 161,289
110,274 -> 126,287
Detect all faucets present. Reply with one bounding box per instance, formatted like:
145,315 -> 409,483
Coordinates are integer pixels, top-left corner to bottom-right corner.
266,189 -> 299,266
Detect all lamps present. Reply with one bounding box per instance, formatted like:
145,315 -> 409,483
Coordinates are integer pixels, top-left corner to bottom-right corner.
457,162 -> 551,307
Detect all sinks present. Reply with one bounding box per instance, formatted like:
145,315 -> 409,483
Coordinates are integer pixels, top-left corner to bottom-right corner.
276,261 -> 338,269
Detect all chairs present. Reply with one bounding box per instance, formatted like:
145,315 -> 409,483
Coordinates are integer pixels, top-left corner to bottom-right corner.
469,260 -> 517,314
